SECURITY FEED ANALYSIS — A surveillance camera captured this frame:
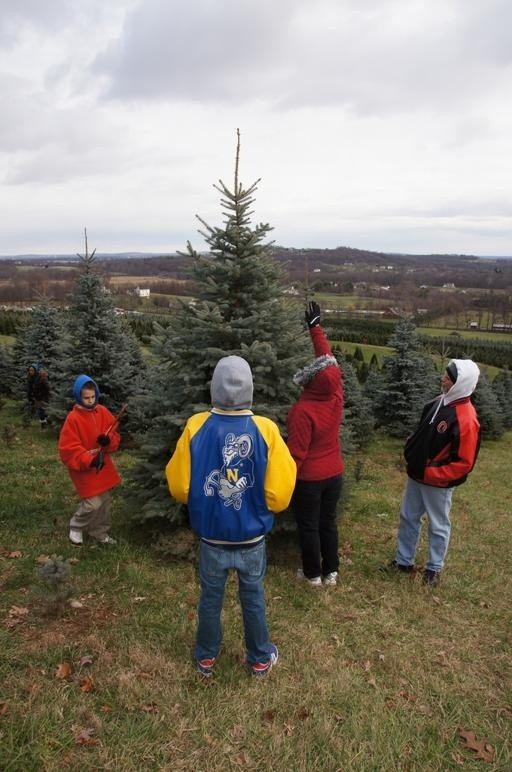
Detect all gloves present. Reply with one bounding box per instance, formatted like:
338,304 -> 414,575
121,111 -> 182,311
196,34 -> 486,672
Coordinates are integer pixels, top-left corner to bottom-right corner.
97,435 -> 110,446
304,301 -> 320,327
91,457 -> 105,469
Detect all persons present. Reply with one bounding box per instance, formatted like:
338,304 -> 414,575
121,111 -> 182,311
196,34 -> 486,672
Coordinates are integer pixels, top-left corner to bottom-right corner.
166,354 -> 297,679
58,373 -> 123,546
286,300 -> 345,588
379,358 -> 482,589
21,363 -> 52,429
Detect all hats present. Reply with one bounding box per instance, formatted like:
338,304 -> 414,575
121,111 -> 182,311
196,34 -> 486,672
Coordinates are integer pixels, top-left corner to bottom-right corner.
447,363 -> 457,384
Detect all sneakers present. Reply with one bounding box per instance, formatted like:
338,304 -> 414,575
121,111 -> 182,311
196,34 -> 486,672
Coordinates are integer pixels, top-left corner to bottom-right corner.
197,657 -> 216,677
297,568 -> 322,587
388,561 -> 414,573
426,569 -> 439,587
323,571 -> 337,585
69,529 -> 83,544
100,536 -> 116,544
250,642 -> 278,676
